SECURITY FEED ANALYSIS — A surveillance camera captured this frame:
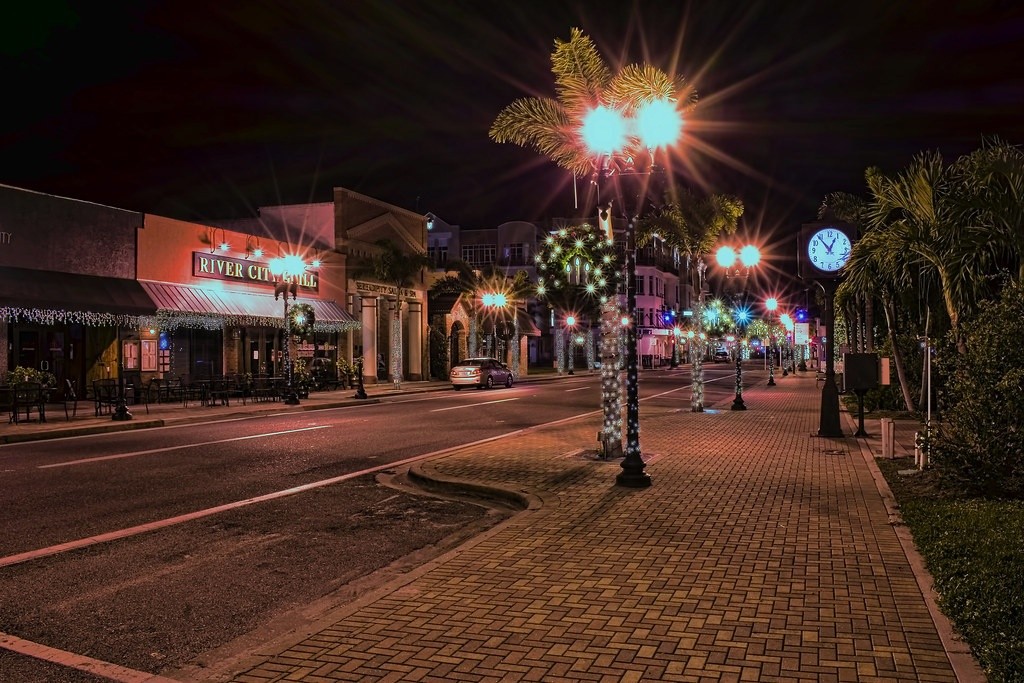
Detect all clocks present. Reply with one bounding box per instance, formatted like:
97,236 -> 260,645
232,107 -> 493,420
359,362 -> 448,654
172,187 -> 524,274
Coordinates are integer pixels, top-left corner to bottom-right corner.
808,228 -> 852,272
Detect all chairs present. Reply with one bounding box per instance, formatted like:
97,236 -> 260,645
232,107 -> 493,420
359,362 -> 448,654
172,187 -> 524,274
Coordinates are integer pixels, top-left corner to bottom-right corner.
0,366 -> 348,425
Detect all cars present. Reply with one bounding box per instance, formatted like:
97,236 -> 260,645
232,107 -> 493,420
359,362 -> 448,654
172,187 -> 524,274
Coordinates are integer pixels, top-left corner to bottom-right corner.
448,358 -> 516,391
713,350 -> 731,363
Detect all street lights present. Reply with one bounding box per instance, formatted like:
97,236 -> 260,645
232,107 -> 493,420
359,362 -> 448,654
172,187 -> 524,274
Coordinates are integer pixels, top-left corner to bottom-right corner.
765,297 -> 778,386
714,242 -> 762,410
566,316 -> 576,375
576,96 -> 685,488
268,255 -> 305,399
780,314 -> 794,376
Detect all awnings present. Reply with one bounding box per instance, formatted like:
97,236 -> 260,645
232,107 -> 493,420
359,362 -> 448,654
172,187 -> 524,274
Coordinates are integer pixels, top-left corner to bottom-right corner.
136,280 -> 362,333
479,307 -> 541,337
0,264 -> 157,329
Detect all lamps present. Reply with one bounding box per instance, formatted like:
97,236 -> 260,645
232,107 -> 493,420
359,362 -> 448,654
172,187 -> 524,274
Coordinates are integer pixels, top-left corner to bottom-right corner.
210,226 -> 320,268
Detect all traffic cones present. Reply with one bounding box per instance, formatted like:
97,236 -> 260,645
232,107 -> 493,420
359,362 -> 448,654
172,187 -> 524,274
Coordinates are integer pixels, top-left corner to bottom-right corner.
482,292 -> 506,359
